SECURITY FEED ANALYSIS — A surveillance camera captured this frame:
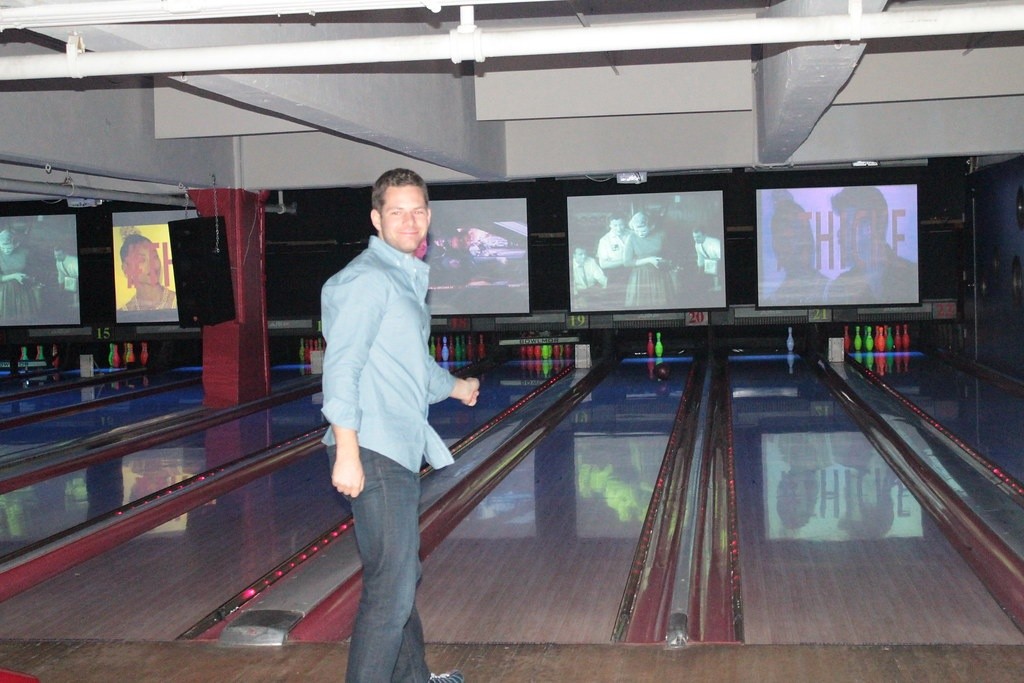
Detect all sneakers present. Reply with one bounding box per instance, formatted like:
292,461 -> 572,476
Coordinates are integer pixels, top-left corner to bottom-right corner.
427,670 -> 464,683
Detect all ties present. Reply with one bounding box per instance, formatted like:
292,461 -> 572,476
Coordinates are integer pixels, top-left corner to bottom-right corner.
582,266 -> 588,287
700,243 -> 709,259
61,261 -> 69,276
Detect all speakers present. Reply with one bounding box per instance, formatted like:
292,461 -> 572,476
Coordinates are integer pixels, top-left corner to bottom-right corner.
168,216 -> 236,329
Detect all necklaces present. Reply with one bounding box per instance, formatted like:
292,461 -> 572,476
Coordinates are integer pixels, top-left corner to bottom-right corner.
135,287 -> 170,310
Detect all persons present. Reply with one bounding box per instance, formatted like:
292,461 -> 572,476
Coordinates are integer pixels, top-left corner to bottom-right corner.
573,208 -> 721,307
115,234 -> 177,311
767,187 -> 919,306
0,228 -> 78,324
321,168 -> 479,683
